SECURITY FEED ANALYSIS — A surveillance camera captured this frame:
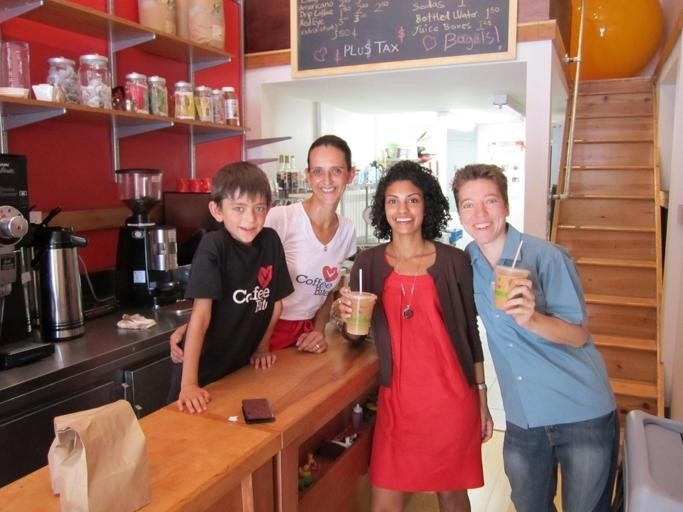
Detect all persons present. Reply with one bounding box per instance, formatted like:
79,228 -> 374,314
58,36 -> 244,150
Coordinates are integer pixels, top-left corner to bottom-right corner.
166,161 -> 295,414
452,164 -> 620,511
331,161 -> 493,511
169,133 -> 357,365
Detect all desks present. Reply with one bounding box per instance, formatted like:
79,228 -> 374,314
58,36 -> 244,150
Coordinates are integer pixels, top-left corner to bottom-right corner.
0,314 -> 381,512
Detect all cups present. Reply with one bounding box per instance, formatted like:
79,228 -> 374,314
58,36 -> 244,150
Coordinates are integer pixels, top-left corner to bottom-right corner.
343,291 -> 377,337
1,40 -> 31,99
491,263 -> 530,312
175,176 -> 212,193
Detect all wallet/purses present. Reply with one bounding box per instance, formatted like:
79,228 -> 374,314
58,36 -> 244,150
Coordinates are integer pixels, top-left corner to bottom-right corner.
241,398 -> 276,423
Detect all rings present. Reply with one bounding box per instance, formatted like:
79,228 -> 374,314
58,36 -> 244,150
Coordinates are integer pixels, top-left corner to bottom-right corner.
338,301 -> 342,304
314,344 -> 320,352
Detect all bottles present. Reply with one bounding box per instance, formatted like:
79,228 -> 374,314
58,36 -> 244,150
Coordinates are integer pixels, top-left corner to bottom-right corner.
137,0 -> 226,52
47,54 -> 81,105
124,71 -> 149,115
351,403 -> 364,431
146,75 -> 168,118
172,80 -> 241,127
275,154 -> 299,195
78,52 -> 113,111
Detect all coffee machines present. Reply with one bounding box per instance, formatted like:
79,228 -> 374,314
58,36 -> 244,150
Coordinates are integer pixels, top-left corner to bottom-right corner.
114,167 -> 184,308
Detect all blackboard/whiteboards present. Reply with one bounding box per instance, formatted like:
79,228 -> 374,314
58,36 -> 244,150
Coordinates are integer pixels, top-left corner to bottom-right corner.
289,0 -> 518,78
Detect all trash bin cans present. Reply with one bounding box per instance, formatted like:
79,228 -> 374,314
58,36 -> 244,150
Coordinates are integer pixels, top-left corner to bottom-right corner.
623,409 -> 683,512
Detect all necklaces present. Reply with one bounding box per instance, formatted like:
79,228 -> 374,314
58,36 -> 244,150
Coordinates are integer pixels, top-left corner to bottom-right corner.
304,210 -> 337,251
394,240 -> 426,319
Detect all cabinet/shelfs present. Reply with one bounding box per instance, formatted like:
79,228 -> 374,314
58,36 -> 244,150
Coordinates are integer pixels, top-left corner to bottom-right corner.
271,186 -> 390,248
0,1 -> 245,188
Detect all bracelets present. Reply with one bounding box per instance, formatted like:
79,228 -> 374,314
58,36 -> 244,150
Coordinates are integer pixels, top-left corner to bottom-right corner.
476,383 -> 486,391
